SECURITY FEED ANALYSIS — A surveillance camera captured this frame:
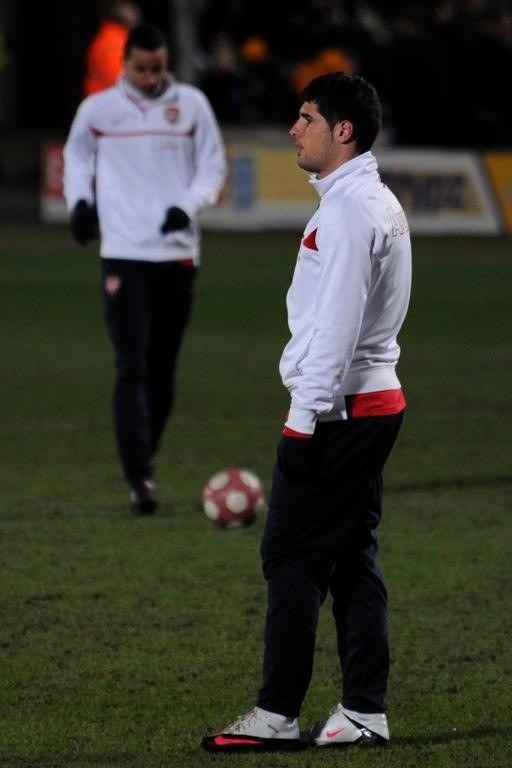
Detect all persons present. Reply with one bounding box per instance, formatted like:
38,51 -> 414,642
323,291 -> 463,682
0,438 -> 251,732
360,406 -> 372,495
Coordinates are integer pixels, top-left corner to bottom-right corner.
84,0 -> 142,95
62,24 -> 227,514
201,72 -> 413,754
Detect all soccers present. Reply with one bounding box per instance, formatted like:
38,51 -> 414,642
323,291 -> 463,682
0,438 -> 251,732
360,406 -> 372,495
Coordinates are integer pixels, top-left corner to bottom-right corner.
204,469 -> 264,528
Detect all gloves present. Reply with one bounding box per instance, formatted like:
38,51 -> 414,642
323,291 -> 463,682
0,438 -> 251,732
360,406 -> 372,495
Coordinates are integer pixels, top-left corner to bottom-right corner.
71,199 -> 100,246
161,208 -> 190,237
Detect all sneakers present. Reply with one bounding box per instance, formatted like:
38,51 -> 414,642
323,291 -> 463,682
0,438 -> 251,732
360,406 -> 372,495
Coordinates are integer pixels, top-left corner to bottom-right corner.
129,479 -> 158,516
202,703 -> 303,752
299,703 -> 390,746
